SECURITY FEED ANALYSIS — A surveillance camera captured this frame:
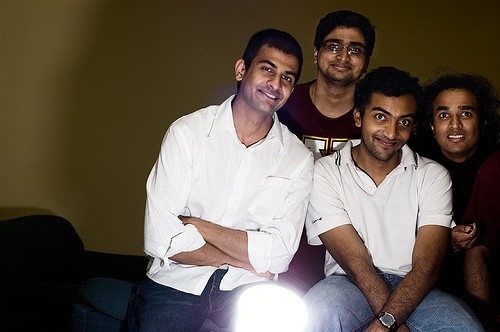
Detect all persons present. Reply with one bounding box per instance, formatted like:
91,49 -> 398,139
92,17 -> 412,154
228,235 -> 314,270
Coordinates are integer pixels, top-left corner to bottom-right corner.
122,8 -> 500,332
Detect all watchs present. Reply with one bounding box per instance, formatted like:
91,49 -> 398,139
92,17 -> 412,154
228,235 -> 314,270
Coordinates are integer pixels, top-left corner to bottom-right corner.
378,312 -> 397,331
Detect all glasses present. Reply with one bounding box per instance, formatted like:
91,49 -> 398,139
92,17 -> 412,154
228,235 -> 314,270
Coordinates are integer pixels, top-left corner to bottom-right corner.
321,41 -> 367,55
